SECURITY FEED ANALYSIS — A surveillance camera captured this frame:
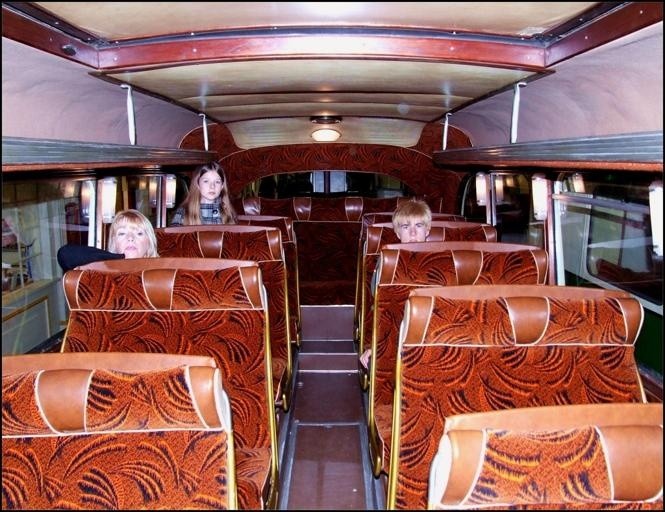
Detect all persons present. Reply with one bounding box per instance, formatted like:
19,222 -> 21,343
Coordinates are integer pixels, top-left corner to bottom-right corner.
359,198 -> 432,371
170,160 -> 239,226
57,208 -> 160,276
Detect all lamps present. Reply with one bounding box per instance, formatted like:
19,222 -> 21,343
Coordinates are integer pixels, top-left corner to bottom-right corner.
649,180 -> 664,257
531,172 -> 548,221
102,176 -> 118,224
165,173 -> 176,209
476,172 -> 488,206
311,128 -> 342,141
310,116 -> 342,124
